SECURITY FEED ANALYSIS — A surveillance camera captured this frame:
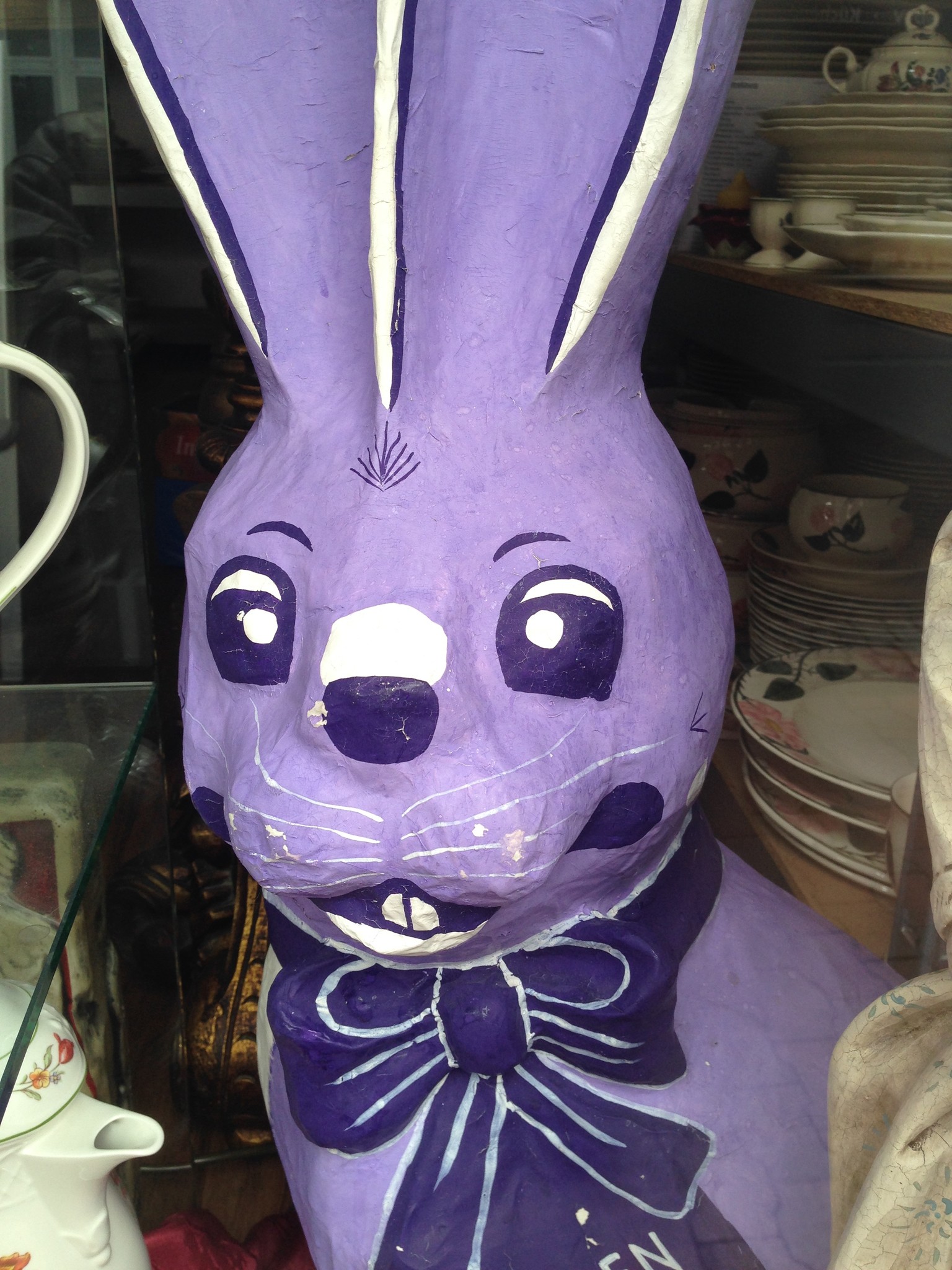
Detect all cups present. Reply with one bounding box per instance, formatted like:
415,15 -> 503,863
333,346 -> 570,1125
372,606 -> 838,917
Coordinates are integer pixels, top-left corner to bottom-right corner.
697,204 -> 757,258
890,774 -> 918,897
742,197 -> 795,267
675,209 -> 698,254
786,196 -> 861,270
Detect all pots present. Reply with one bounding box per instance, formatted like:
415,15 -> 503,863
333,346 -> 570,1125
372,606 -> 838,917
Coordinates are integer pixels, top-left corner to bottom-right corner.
655,389 -> 815,630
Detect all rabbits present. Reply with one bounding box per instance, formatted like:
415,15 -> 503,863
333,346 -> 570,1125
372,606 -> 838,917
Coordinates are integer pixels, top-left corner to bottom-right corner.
91,0 -> 909,1270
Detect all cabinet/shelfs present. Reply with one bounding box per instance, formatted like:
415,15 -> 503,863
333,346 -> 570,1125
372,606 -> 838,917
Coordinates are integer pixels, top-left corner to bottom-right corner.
648,250 -> 951,986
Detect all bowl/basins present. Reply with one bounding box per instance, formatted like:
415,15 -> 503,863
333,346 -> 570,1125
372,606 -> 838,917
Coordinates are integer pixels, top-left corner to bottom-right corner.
788,474 -> 914,566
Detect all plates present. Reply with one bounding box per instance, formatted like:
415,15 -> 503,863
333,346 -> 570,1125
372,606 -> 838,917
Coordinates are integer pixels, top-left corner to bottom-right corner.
816,272 -> 952,291
681,342 -> 767,387
730,524 -> 934,667
853,439 -> 950,520
739,729 -> 889,837
729,646 -> 921,805
736,0 -> 907,72
842,198 -> 952,235
740,756 -> 898,899
784,226 -> 952,271
692,72 -> 952,203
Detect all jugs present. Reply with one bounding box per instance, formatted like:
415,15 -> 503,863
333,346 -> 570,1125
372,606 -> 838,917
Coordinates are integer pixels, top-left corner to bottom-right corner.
0,977 -> 165,1270
820,6 -> 952,91
